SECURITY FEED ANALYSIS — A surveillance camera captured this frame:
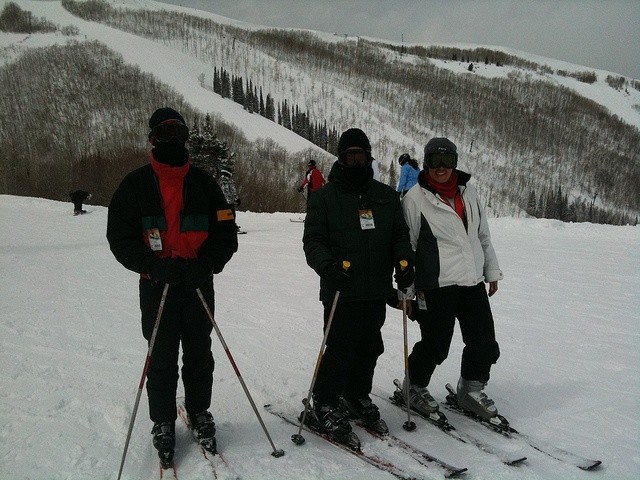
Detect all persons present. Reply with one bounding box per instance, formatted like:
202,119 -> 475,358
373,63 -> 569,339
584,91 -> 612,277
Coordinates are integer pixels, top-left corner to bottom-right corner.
302,128 -> 415,432
394,153 -> 422,197
398,138 -> 506,422
105,110 -> 240,466
69,190 -> 92,213
297,159 -> 326,193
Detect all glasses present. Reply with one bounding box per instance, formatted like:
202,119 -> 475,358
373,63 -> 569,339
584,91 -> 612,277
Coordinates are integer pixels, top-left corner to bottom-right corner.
425,153 -> 457,168
153,125 -> 189,142
338,149 -> 372,168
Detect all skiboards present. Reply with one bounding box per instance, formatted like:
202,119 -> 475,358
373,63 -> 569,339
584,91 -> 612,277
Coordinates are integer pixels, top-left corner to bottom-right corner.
160,396 -> 243,480
370,385 -> 601,470
264,404 -> 468,480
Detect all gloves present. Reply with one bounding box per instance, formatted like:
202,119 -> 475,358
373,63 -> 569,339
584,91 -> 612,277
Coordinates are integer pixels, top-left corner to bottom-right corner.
328,254 -> 350,291
184,262 -> 207,288
150,262 -> 181,285
394,256 -> 414,287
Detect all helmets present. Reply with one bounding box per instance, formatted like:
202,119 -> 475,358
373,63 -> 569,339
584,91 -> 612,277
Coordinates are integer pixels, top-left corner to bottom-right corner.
399,153 -> 409,165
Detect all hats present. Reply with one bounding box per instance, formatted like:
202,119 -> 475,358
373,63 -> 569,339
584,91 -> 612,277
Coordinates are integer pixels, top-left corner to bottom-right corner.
337,129 -> 371,155
149,107 -> 186,130
424,138 -> 456,153
307,160 -> 316,166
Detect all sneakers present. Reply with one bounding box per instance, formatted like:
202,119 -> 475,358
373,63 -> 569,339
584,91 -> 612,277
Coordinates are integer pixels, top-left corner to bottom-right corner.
151,422 -> 175,452
457,375 -> 498,421
187,411 -> 216,440
403,377 -> 439,416
312,393 -> 352,436
340,395 -> 380,422
